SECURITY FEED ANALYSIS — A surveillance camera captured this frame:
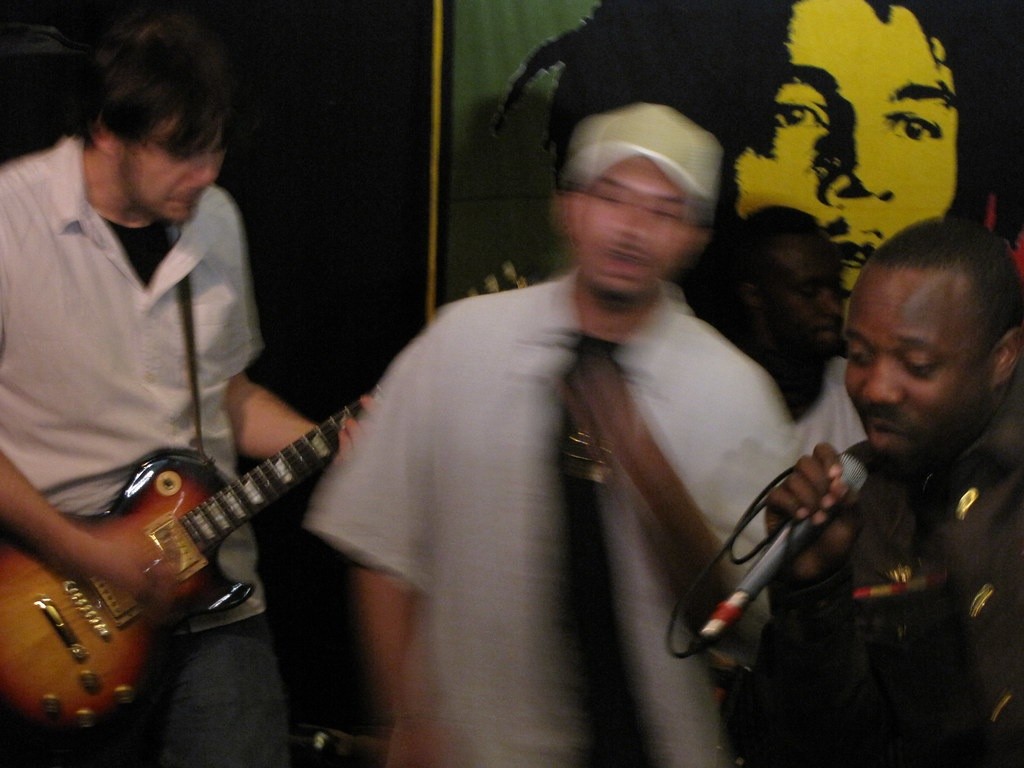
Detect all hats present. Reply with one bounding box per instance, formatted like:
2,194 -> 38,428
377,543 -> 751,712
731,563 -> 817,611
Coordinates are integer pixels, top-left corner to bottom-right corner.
561,99 -> 723,203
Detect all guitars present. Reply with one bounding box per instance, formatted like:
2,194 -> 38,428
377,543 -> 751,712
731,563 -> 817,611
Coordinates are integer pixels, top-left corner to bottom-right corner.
0,258 -> 537,752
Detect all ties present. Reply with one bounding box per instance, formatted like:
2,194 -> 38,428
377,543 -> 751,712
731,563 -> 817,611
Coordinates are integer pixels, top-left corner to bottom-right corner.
560,336 -> 657,768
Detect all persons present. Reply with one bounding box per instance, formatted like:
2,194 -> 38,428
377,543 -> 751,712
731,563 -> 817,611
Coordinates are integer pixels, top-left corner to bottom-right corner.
301,101 -> 798,768
702,207 -> 1024,766
0,1 -> 379,768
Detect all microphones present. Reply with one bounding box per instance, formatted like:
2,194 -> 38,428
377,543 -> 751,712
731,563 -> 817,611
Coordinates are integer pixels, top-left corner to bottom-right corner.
687,453 -> 866,657
